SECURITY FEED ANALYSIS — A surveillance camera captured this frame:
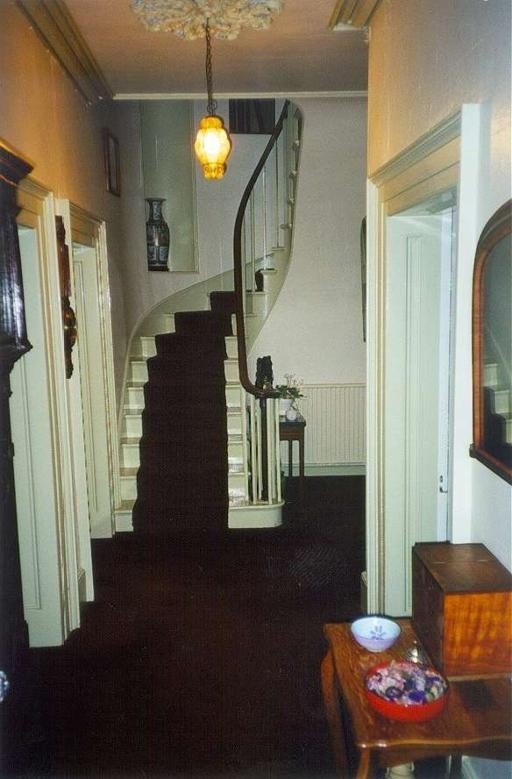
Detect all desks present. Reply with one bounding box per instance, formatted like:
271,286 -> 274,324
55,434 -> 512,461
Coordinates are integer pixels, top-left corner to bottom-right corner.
319,617 -> 512,779
247,407 -> 306,480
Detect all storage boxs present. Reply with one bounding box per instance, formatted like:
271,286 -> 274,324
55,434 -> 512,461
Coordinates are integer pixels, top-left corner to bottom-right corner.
410,543 -> 512,681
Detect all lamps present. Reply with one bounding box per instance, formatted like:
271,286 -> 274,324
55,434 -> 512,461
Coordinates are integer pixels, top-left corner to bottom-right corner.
194,17 -> 231,180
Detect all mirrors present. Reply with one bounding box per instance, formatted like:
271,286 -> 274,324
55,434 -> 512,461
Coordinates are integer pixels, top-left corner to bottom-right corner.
469,198 -> 512,486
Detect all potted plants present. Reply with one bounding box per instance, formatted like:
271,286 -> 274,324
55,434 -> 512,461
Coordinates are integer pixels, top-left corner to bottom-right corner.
275,385 -> 303,416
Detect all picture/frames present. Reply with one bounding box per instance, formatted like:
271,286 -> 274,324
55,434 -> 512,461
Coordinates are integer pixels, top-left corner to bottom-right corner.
103,126 -> 120,197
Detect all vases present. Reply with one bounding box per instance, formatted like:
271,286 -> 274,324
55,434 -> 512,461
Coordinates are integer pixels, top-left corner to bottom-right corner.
145,198 -> 170,271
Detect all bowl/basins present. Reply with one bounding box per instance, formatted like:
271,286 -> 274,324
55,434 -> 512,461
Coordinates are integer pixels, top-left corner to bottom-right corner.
361,662 -> 450,724
350,616 -> 402,651
